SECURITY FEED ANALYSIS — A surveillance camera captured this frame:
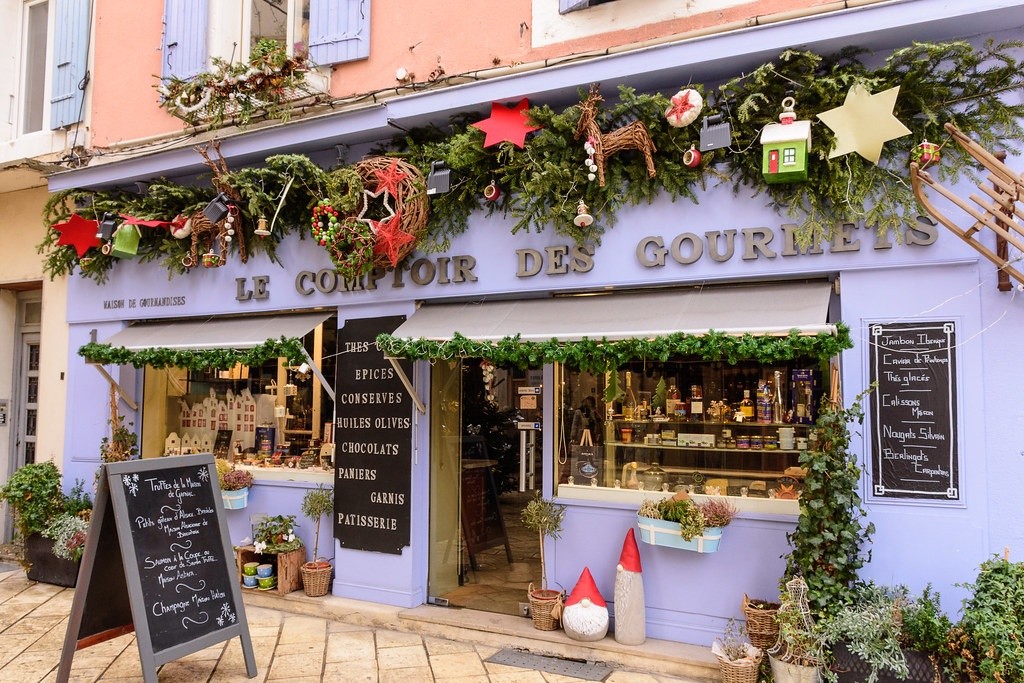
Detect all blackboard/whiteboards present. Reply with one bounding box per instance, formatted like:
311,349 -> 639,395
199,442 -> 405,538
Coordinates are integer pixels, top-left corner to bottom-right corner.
446,436 -> 507,552
69,454 -> 244,660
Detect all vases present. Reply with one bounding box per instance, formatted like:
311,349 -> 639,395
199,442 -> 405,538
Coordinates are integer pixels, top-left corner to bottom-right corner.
268,534 -> 284,544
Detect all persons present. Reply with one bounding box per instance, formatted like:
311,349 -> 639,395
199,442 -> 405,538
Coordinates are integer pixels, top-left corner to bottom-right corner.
570,396 -> 603,468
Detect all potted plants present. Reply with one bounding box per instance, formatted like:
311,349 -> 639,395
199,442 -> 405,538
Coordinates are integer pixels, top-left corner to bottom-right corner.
519,489 -> 566,600
0,460 -> 93,588
215,459 -> 253,509
637,497 -> 736,553
300,481 -> 335,568
765,575 -> 826,683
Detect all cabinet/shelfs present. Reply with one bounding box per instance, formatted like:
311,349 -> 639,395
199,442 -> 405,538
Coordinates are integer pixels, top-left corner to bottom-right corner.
605,361 -> 821,501
198,362 -> 312,454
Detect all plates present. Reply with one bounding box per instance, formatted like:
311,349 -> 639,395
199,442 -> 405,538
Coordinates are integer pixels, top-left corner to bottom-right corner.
241,584 -> 276,591
254,574 -> 274,579
242,574 -> 257,578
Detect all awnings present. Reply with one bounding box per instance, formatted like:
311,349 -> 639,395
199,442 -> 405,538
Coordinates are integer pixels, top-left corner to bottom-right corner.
377,277 -> 854,412
78,309 -> 336,409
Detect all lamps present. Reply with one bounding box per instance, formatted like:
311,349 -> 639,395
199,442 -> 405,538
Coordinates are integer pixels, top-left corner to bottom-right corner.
700,114 -> 733,151
204,193 -> 249,222
95,212 -> 127,241
427,161 -> 475,193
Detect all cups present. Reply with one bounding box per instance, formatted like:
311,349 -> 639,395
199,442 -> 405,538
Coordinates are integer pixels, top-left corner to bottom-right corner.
621,428 -> 632,443
674,402 -> 686,420
243,562 -> 278,588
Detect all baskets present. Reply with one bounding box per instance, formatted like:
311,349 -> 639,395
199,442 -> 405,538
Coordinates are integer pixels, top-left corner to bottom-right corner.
300,563 -> 332,597
743,592 -> 779,664
716,645 -> 761,683
527,583 -> 567,631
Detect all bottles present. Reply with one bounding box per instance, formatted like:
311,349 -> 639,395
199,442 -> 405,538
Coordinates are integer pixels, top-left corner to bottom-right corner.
641,463 -> 668,491
308,437 -> 332,468
666,377 -> 682,422
795,380 -> 805,423
234,439 -> 242,453
625,460 -> 639,490
740,390 -> 755,422
622,371 -> 636,422
640,401 -> 650,420
761,369 -> 782,423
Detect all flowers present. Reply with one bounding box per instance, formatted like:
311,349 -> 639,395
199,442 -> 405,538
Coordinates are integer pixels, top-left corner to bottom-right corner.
253,513 -> 299,554
